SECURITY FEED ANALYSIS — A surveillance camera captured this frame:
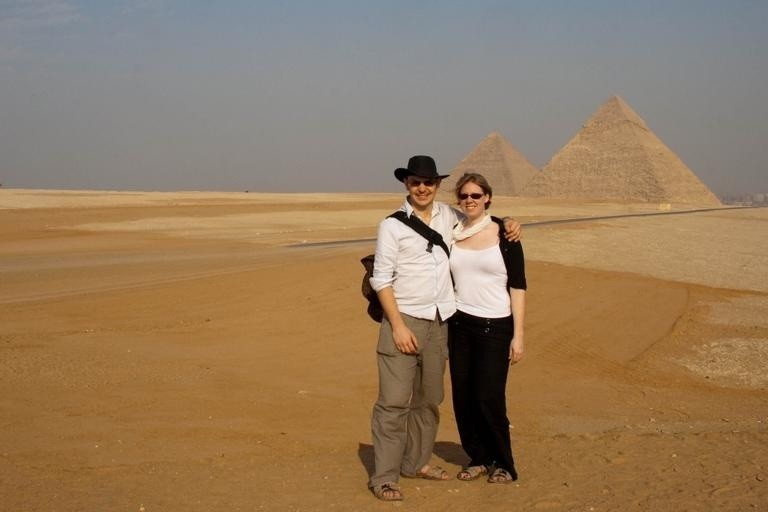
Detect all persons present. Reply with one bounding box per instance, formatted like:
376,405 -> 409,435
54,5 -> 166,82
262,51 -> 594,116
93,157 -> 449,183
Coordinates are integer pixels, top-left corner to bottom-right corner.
445,172 -> 529,484
368,156 -> 522,499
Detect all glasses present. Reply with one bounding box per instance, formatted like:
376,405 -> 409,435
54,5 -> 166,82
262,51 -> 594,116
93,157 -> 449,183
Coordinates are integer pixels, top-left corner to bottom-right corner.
410,181 -> 432,186
459,194 -> 483,199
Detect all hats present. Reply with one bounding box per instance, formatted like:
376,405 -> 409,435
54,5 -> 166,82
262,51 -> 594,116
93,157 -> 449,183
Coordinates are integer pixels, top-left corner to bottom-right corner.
394,156 -> 450,183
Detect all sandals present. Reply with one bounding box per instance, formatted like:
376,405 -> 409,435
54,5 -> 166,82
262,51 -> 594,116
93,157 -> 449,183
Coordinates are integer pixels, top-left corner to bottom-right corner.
457,465 -> 495,480
489,468 -> 513,483
373,481 -> 404,500
400,464 -> 448,480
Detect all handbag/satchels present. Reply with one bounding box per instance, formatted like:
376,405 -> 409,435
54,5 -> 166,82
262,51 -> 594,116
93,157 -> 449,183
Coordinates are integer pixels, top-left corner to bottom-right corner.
361,255 -> 384,322
